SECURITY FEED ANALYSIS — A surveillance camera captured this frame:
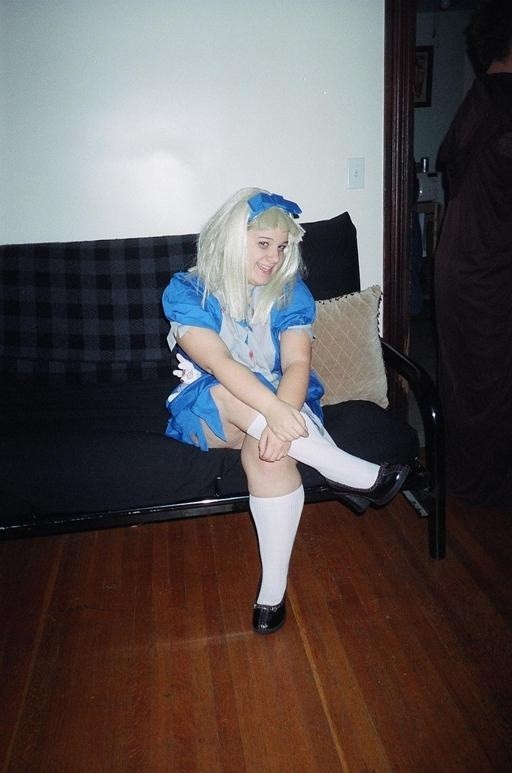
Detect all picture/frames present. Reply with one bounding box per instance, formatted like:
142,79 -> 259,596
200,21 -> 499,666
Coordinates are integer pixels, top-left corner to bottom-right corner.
411,43 -> 435,110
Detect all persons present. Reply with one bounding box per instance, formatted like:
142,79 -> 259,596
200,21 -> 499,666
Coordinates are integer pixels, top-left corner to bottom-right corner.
434,1 -> 512,508
161,186 -> 410,637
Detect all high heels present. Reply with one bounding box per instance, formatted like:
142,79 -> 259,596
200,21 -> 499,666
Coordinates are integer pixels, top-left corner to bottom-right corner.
251,573 -> 287,634
326,462 -> 410,515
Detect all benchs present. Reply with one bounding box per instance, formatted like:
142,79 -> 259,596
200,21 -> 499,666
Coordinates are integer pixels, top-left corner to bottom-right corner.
1,209 -> 455,565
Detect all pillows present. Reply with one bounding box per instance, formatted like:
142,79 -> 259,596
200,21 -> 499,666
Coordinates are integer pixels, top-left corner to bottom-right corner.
307,277 -> 388,412
